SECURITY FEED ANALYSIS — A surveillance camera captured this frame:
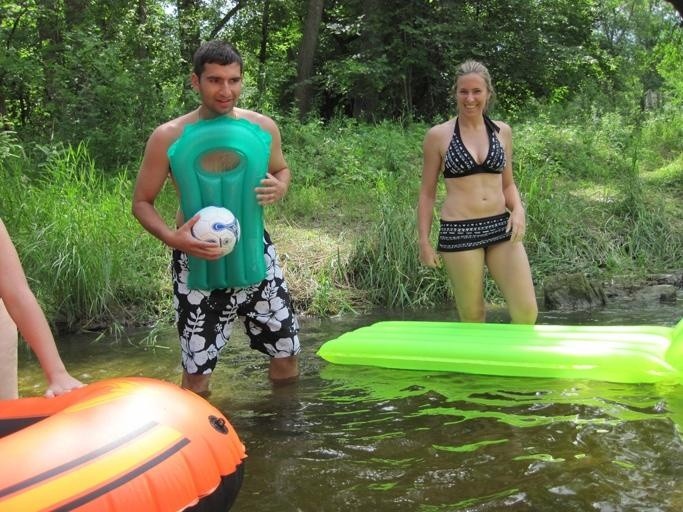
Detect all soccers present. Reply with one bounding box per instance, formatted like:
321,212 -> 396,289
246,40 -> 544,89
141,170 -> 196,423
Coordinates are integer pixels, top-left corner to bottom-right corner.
190,206 -> 241,258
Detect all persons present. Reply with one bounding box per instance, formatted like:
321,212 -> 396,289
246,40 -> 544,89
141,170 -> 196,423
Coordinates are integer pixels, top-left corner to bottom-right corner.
129,39 -> 300,392
0,215 -> 87,400
417,61 -> 538,326
